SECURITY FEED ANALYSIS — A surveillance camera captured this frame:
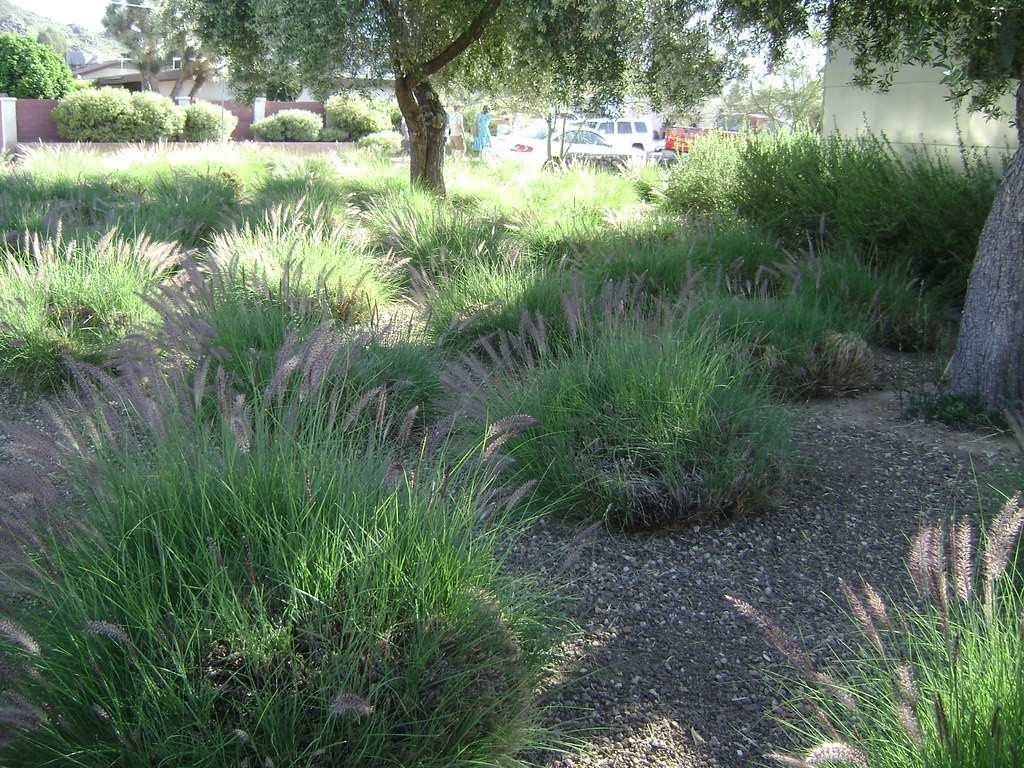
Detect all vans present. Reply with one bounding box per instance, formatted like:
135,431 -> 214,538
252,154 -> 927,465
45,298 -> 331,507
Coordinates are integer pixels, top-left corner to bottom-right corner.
566,116 -> 652,153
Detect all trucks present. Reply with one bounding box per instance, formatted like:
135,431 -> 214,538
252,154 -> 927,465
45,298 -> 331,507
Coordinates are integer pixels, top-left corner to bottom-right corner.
662,112 -> 785,156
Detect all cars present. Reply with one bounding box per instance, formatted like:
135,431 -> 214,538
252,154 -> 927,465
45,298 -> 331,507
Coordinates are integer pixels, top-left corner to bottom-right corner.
481,123 -> 642,179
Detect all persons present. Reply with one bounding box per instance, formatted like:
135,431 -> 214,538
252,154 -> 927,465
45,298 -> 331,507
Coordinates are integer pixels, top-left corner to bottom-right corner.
400,114 -> 412,156
450,104 -> 468,162
470,104 -> 492,158
